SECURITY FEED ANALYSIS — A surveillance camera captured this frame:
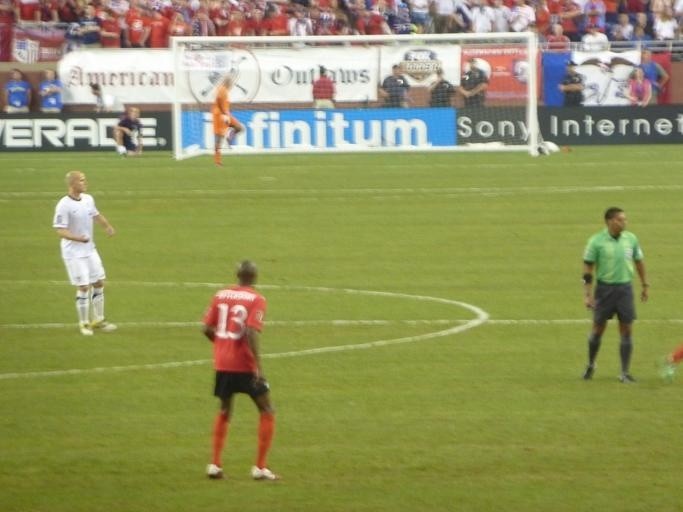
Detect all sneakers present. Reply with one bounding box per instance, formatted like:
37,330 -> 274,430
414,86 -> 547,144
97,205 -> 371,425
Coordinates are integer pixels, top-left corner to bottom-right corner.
205,464 -> 223,478
79,319 -> 116,336
252,466 -> 281,481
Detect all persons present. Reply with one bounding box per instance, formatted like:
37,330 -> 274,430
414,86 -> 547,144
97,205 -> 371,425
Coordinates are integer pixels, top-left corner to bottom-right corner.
638,50 -> 670,105
211,78 -> 244,167
113,106 -> 144,157
557,60 -> 586,106
313,67 -> 335,108
38,69 -> 65,115
623,68 -> 653,108
428,68 -> 456,107
582,207 -> 650,384
1,0 -> 683,52
202,260 -> 283,480
664,342 -> 683,364
459,59 -> 490,107
379,65 -> 411,108
2,68 -> 33,114
52,171 -> 117,336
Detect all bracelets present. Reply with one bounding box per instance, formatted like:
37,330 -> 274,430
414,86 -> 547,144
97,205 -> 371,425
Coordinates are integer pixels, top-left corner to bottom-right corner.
642,283 -> 649,288
582,273 -> 593,286
